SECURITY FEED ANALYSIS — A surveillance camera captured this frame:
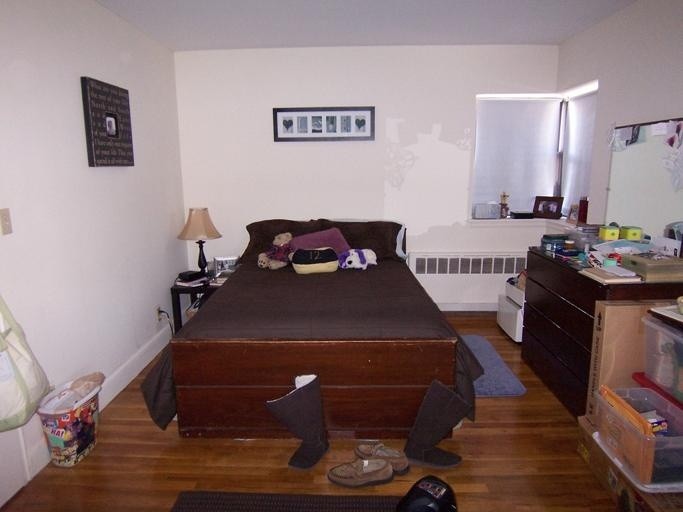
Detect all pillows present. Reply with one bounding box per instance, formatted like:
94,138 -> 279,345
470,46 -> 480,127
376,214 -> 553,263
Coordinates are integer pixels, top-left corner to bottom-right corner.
235,220 -> 315,267
316,218 -> 407,262
288,227 -> 349,256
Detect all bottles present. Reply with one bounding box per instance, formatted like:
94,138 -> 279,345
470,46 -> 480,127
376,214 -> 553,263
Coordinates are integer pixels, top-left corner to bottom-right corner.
499,203 -> 509,218
575,196 -> 589,225
613,248 -> 621,265
563,240 -> 575,249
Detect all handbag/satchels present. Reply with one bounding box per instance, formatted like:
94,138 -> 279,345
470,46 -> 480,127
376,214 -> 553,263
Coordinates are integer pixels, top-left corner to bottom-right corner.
0,294 -> 51,432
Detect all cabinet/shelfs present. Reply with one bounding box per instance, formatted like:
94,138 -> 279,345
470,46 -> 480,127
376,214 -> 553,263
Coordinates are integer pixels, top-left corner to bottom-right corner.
520,245 -> 682,423
495,281 -> 525,343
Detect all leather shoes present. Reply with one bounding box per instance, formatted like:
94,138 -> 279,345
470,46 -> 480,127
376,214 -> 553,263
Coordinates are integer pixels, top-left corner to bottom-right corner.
327,455 -> 393,488
354,442 -> 408,475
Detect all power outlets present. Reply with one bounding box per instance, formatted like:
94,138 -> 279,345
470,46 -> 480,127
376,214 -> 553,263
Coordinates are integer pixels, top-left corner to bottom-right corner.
155,306 -> 162,322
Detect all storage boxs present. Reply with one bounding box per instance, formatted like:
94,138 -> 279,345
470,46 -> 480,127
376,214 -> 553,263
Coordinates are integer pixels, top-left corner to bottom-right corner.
573,298 -> 682,512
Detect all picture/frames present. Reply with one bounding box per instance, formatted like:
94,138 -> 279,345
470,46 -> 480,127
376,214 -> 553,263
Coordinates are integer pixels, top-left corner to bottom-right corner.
532,195 -> 564,220
271,106 -> 376,143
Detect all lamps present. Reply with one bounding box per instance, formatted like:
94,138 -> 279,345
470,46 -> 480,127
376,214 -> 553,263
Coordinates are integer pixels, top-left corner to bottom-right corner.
176,207 -> 221,278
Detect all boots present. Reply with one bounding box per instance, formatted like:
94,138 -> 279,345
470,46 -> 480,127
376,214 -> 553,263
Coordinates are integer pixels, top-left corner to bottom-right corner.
264,373 -> 329,468
404,379 -> 472,465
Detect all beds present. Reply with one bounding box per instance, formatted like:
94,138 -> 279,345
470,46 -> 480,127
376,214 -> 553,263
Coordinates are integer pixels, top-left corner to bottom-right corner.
137,217 -> 484,439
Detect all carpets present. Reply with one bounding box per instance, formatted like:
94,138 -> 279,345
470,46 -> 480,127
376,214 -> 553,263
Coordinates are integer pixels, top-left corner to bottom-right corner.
457,333 -> 526,398
168,492 -> 402,512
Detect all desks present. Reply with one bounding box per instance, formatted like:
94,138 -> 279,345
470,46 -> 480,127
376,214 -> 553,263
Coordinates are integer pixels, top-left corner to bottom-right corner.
170,278 -> 226,335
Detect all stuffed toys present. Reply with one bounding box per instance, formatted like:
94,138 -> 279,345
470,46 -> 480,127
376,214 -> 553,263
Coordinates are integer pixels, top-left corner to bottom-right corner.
258,231 -> 296,270
338,248 -> 379,270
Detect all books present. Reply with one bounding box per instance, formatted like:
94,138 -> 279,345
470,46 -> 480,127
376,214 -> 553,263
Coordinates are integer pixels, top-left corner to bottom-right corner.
576,250 -> 683,286
173,269 -> 233,288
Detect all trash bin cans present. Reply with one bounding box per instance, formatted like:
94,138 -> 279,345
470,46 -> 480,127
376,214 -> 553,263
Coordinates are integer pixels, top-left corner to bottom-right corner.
36,376 -> 102,467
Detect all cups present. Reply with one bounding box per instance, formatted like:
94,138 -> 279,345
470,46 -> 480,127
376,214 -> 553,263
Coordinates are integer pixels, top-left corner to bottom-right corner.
603,259 -> 616,268
